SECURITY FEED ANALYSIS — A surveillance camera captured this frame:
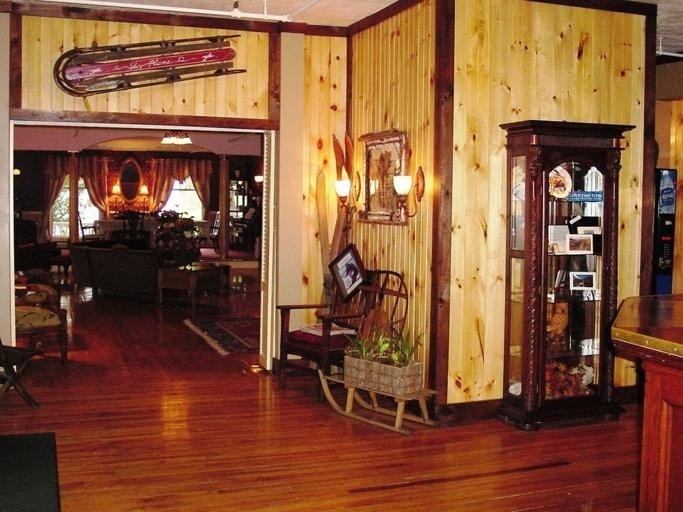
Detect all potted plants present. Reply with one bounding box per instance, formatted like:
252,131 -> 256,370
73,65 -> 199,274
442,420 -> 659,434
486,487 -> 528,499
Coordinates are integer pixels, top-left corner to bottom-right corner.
344,326 -> 424,395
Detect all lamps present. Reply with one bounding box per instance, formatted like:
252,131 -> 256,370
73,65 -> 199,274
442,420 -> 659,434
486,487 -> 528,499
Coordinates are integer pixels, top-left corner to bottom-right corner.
112,185 -> 121,214
334,172 -> 360,213
393,167 -> 425,218
161,130 -> 193,145
139,185 -> 150,213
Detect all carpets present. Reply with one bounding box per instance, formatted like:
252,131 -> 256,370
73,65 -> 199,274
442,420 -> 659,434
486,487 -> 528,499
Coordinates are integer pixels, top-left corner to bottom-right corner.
0,432 -> 60,512
184,318 -> 260,355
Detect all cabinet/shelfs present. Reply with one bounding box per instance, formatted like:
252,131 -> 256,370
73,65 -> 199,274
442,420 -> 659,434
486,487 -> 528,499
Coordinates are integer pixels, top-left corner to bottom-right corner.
498,120 -> 636,430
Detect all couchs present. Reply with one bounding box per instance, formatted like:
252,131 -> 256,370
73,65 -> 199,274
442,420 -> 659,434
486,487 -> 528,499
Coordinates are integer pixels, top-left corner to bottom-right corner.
71,241 -> 160,301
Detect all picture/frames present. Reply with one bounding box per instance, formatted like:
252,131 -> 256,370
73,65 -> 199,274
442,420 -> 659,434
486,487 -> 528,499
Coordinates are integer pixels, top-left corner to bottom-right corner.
356,129 -> 409,225
328,244 -> 370,304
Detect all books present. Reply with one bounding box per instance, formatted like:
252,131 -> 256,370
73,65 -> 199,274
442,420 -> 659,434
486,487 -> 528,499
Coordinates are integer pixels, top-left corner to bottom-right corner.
300,322 -> 358,337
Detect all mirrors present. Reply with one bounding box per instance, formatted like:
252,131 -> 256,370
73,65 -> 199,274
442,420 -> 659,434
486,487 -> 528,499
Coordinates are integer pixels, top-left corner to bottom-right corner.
119,157 -> 143,202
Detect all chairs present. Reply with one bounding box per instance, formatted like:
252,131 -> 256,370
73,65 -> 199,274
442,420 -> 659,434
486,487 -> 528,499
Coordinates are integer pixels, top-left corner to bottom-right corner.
80,219 -> 104,241
44,254 -> 70,283
16,305 -> 67,362
276,270 -> 379,404
196,210 -> 220,254
0,345 -> 40,408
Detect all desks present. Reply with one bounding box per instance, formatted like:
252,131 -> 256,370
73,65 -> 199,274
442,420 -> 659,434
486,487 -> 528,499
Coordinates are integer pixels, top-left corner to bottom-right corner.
158,261 -> 231,312
317,368 -> 439,435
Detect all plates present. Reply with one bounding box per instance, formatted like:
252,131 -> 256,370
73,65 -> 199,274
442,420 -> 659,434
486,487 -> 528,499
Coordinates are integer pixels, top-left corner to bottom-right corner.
549,165 -> 573,198
